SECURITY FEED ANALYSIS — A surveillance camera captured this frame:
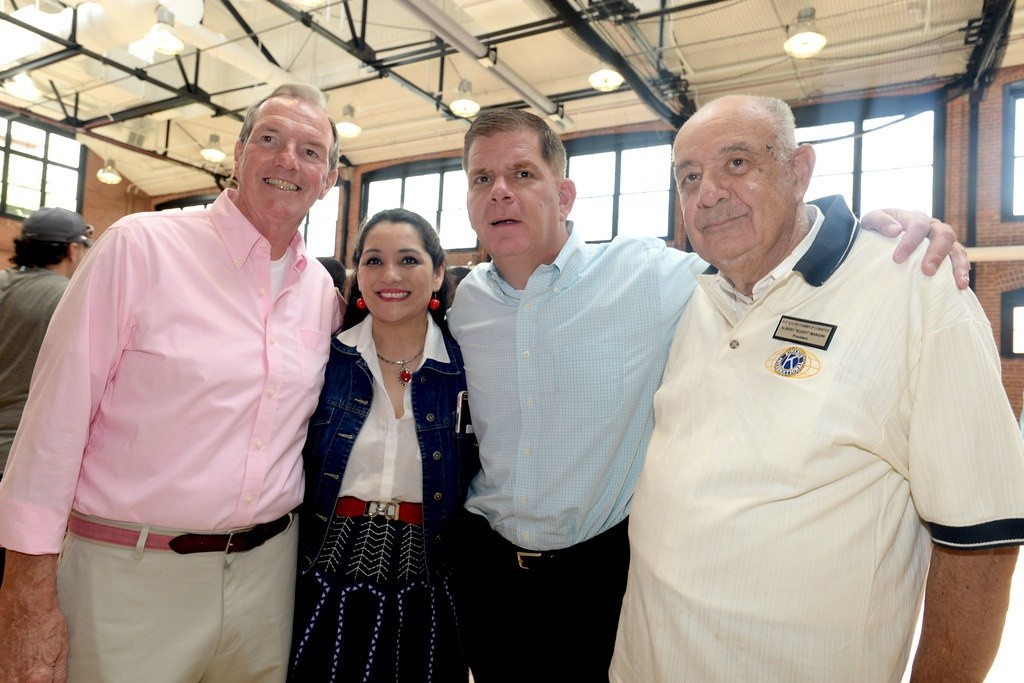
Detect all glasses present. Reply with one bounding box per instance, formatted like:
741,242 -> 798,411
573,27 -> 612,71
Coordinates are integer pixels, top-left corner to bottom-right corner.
67,224 -> 94,241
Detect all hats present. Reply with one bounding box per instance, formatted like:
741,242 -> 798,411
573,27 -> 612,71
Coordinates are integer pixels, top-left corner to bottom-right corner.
21,208 -> 92,247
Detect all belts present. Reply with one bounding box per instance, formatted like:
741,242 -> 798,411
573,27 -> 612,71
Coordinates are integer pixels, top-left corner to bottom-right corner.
69,513 -> 293,555
337,495 -> 424,527
477,518 -> 627,570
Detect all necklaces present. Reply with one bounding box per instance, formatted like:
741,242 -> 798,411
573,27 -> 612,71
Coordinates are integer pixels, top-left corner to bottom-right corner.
377,347 -> 425,387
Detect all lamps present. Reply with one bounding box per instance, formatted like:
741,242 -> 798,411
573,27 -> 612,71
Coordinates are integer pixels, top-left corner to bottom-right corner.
199,132 -> 227,164
782,9 -> 827,56
335,104 -> 363,139
128,3 -> 184,67
588,58 -> 626,92
449,79 -> 480,120
96,157 -> 123,186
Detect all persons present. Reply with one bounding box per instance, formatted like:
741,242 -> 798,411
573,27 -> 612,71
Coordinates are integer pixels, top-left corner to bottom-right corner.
0,82 -> 350,683
608,95 -> 1024,683
443,110 -> 973,683
283,209 -> 478,683
